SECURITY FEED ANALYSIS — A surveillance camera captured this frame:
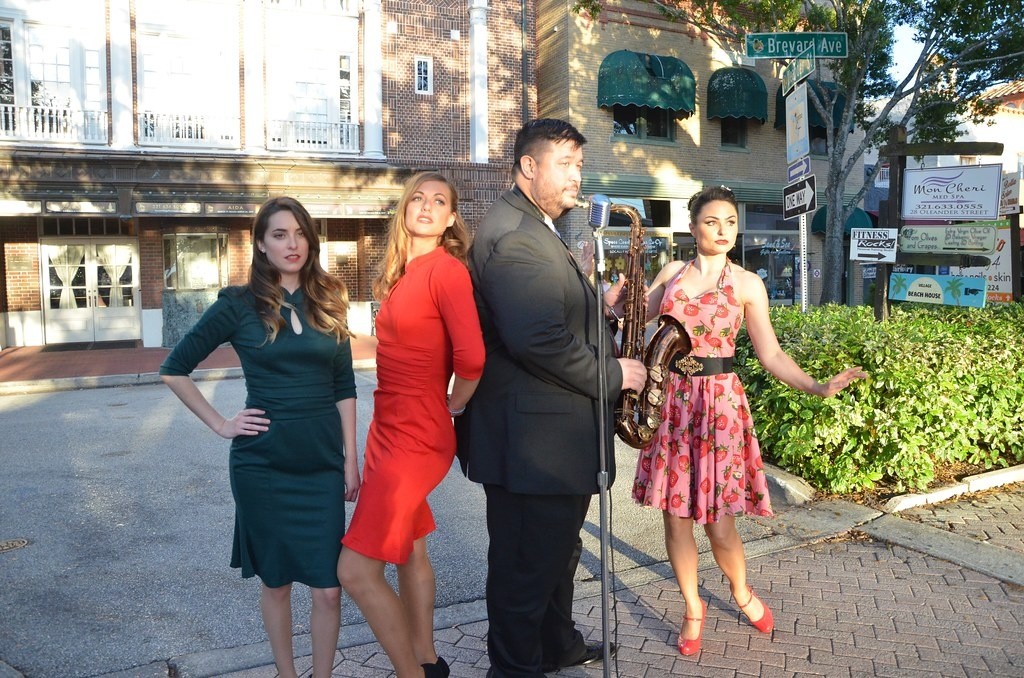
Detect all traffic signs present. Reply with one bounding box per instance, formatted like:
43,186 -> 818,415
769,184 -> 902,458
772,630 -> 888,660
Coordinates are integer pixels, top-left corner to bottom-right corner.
850,227 -> 898,266
781,174 -> 818,221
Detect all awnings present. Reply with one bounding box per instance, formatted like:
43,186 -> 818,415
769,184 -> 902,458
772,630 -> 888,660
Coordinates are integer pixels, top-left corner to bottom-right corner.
774,81 -> 854,134
706,67 -> 770,122
810,205 -> 950,235
596,49 -> 698,116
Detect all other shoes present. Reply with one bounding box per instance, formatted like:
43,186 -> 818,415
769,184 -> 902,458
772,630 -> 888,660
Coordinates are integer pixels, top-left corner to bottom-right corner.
420,657 -> 450,678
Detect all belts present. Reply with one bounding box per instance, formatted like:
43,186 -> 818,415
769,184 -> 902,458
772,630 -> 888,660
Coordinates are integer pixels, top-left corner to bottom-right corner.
669,356 -> 733,376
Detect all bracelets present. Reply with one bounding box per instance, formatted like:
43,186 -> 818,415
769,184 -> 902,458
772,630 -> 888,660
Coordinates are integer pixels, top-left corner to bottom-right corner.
447,393 -> 467,416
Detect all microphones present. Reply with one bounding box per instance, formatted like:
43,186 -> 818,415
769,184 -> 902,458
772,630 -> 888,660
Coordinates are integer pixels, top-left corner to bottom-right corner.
588,194 -> 611,228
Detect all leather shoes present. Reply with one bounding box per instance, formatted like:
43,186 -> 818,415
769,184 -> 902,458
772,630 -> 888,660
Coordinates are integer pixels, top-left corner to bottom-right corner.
678,598 -> 705,655
730,584 -> 773,634
565,642 -> 616,666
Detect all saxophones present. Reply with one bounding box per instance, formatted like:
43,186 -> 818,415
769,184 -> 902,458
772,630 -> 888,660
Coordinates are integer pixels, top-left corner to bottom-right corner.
574,198 -> 692,450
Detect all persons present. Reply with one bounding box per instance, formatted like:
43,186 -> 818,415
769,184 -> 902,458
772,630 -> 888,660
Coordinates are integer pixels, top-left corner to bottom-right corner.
454,118 -> 648,678
159,197 -> 361,678
618,185 -> 865,656
336,172 -> 487,678
